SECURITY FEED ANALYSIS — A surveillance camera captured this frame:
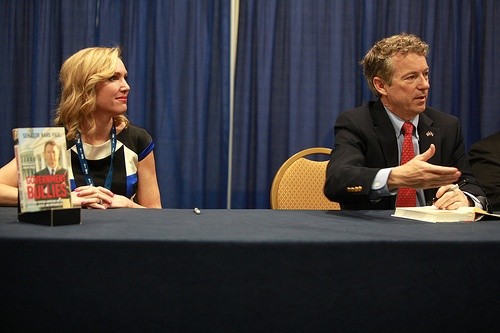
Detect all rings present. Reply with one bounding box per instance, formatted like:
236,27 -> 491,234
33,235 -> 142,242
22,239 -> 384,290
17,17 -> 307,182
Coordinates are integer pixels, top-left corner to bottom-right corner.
98,198 -> 103,205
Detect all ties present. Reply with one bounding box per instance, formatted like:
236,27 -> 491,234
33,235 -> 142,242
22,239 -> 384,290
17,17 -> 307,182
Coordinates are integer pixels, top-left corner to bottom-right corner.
394,122 -> 416,207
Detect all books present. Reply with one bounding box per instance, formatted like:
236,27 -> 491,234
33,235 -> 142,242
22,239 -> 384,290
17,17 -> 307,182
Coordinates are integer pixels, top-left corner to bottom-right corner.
391,203 -> 500,225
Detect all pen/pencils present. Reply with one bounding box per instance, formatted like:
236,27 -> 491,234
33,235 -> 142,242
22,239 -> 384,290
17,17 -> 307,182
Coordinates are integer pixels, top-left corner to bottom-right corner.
193,207 -> 200,214
433,178 -> 468,201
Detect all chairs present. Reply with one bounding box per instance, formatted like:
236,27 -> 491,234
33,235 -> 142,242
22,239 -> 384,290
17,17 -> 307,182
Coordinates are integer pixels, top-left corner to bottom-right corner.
270,145 -> 341,211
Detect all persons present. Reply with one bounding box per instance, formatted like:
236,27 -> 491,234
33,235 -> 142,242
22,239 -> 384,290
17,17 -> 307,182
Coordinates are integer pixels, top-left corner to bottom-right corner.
322,34 -> 493,214
35,140 -> 71,200
12,127 -> 73,214
0,46 -> 162,210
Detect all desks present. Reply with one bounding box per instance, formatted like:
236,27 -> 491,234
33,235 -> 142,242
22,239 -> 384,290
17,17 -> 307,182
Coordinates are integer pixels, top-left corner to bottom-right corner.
0,207 -> 500,333
